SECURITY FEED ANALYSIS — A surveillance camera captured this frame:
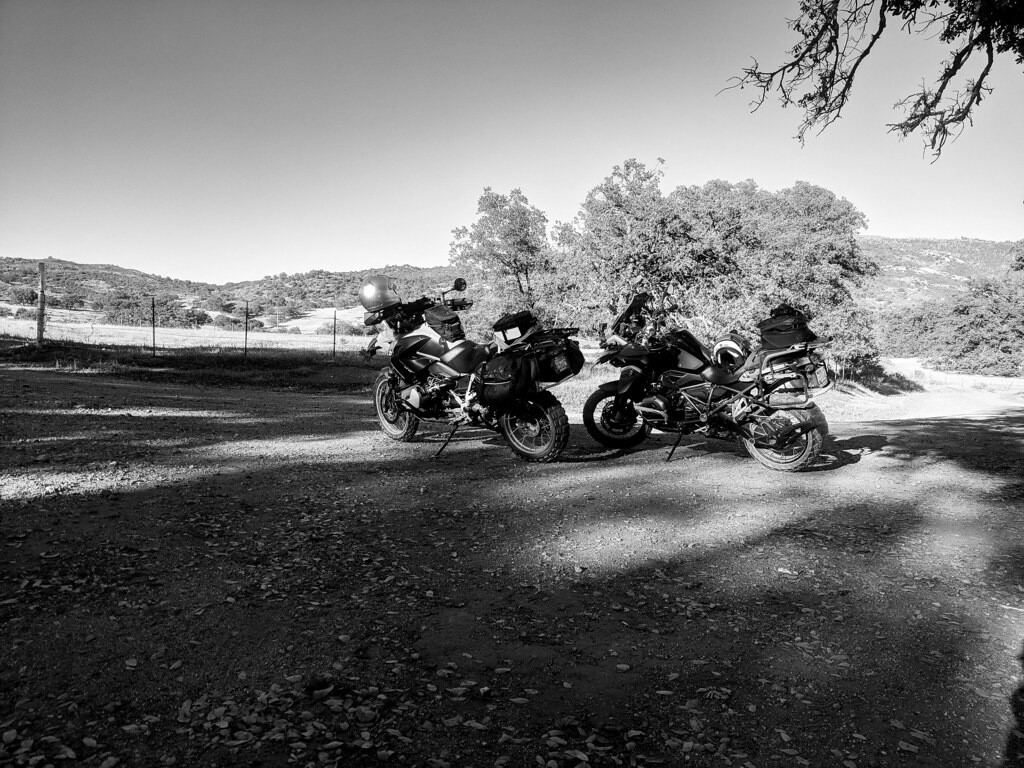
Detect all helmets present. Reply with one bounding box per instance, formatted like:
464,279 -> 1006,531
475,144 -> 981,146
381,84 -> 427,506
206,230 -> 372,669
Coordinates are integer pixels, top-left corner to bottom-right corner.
357,273 -> 401,312
710,332 -> 750,374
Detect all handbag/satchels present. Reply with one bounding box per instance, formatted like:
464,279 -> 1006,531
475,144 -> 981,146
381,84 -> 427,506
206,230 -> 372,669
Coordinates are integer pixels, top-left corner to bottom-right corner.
756,303 -> 818,349
524,328 -> 585,383
475,345 -> 532,409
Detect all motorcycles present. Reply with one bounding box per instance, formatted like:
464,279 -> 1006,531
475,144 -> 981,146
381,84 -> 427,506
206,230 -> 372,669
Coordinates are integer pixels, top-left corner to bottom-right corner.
582,290 -> 837,473
364,278 -> 580,462
